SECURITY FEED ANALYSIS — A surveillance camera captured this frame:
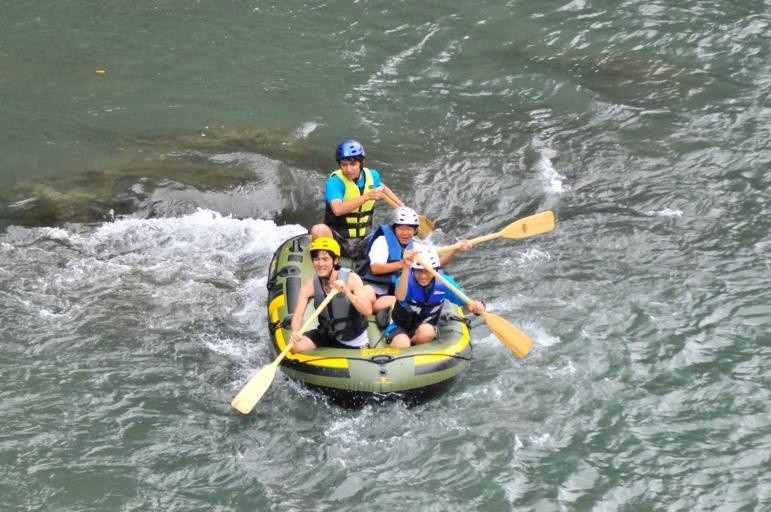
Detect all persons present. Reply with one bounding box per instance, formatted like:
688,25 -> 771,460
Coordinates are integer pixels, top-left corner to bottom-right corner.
299,223 -> 355,289
354,206 -> 473,317
286,237 -> 374,356
383,245 -> 486,350
310,138 -> 408,261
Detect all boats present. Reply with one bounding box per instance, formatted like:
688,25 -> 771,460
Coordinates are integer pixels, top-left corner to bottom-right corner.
266,233 -> 474,411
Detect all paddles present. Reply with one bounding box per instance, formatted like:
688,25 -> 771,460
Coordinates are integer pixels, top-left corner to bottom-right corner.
230,284 -> 339,415
404,250 -> 534,359
377,190 -> 436,240
436,210 -> 555,256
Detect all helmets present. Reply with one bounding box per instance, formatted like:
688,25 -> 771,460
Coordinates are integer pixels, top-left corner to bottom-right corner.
335,139 -> 366,162
409,245 -> 440,272
308,236 -> 340,257
387,206 -> 420,227
